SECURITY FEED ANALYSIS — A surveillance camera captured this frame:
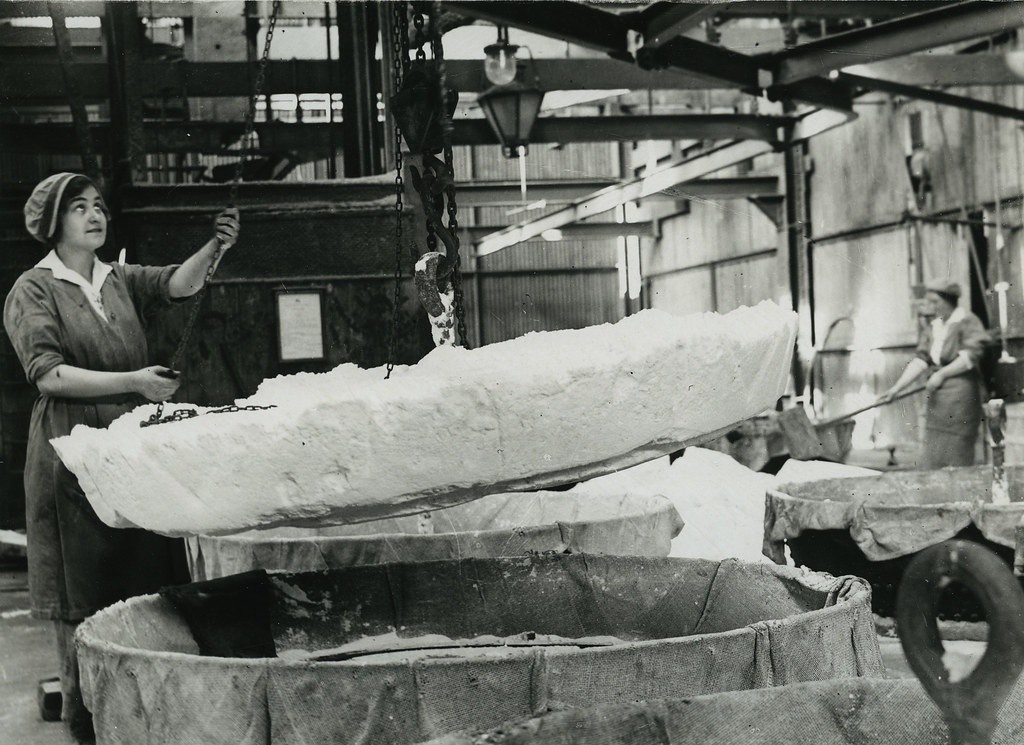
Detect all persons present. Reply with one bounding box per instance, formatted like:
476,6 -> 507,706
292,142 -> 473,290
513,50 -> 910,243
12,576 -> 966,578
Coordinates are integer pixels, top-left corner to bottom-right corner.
3,173 -> 241,745
876,280 -> 992,471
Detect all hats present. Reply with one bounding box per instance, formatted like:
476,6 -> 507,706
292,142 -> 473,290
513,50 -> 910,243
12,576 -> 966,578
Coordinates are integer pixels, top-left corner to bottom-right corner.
927,279 -> 960,298
24,170 -> 112,243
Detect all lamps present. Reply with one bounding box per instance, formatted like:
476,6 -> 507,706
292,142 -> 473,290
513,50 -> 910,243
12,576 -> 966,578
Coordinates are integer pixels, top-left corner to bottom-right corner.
476,80 -> 546,161
